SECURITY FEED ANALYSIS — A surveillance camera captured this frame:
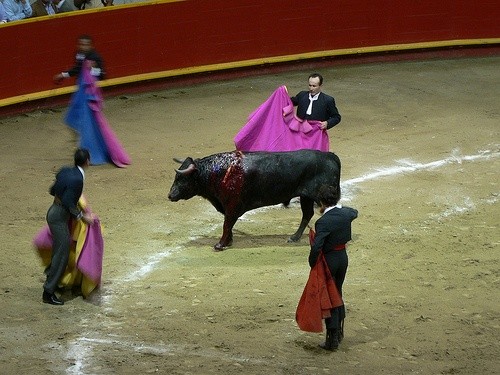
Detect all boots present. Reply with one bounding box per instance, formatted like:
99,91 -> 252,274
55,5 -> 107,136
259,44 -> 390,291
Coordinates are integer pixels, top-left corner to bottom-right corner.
336,320 -> 344,340
317,326 -> 338,351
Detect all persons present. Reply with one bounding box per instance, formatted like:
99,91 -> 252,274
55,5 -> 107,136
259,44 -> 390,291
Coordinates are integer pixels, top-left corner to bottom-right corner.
39,148 -> 97,306
307,183 -> 359,350
282,72 -> 342,131
1,0 -> 126,26
53,34 -> 105,143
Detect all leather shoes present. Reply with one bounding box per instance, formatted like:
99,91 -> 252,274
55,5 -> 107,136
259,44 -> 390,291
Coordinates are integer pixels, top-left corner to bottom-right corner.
43,292 -> 64,305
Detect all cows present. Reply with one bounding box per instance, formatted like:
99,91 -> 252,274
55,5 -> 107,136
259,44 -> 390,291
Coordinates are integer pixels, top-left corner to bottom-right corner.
168,149 -> 341,252
